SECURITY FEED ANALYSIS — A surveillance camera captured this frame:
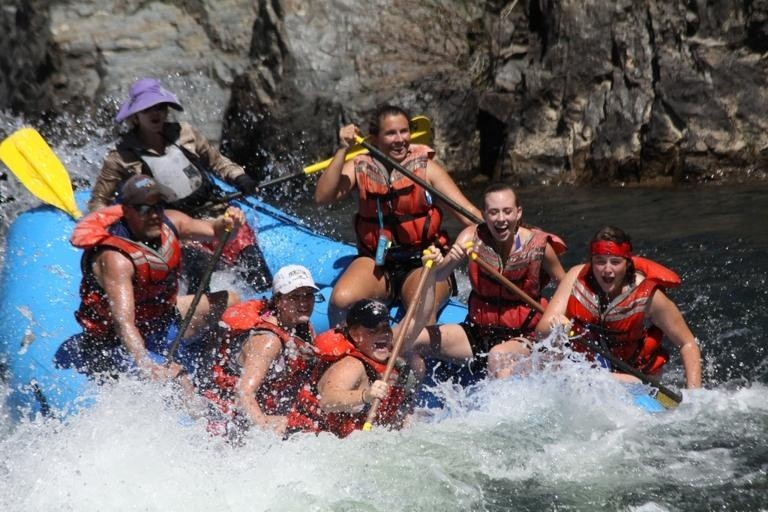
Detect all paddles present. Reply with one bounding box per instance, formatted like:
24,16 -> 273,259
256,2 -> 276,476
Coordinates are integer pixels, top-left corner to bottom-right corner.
461,242 -> 682,410
0,128 -> 85,224
186,115 -> 433,217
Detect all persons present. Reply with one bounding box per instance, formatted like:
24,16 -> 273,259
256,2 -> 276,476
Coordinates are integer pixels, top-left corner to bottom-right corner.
410,183 -> 567,380
74,174 -> 246,397
204,265 -> 321,441
535,227 -> 702,391
86,79 -> 272,295
282,246 -> 444,440
314,105 -> 486,327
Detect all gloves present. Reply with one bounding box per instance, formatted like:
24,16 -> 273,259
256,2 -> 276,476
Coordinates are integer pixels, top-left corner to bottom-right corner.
233,174 -> 262,198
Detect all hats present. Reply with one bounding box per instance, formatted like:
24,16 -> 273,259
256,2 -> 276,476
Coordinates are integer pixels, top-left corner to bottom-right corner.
116,78 -> 184,123
346,298 -> 390,327
120,174 -> 169,205
271,264 -> 320,295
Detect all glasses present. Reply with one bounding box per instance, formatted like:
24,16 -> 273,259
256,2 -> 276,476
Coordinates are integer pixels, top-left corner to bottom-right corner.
124,203 -> 165,219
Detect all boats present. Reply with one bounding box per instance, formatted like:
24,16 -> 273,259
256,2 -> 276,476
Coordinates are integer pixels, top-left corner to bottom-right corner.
1,167 -> 672,429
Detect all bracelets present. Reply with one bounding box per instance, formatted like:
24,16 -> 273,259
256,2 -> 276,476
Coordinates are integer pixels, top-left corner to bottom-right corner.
361,389 -> 371,405
